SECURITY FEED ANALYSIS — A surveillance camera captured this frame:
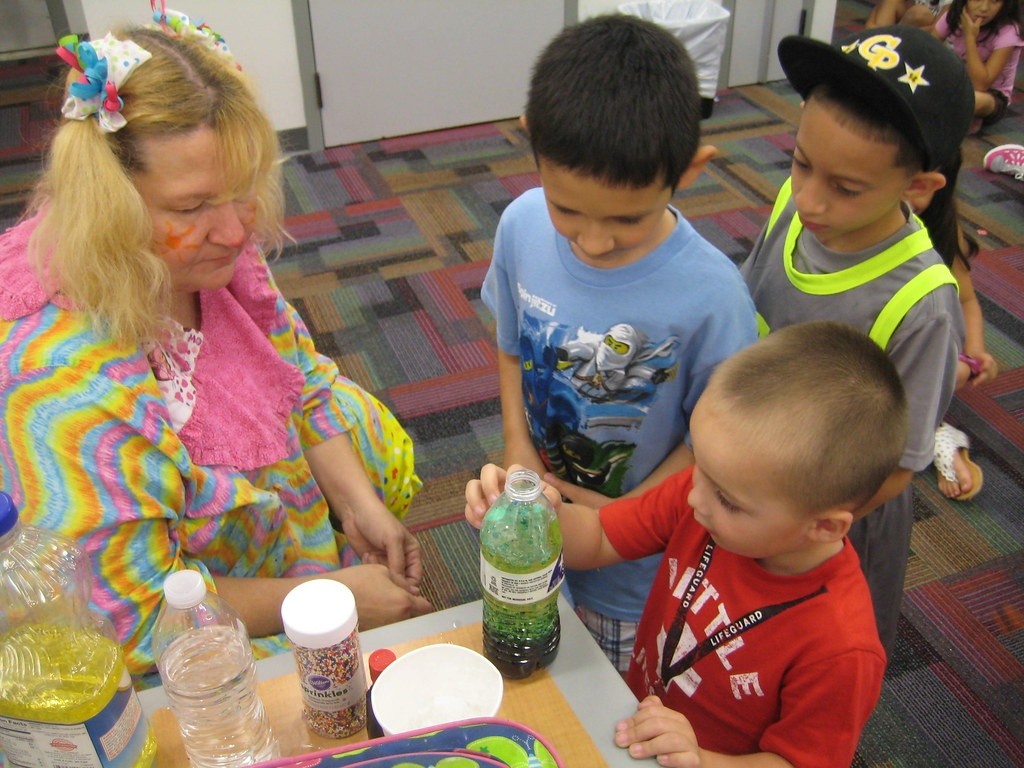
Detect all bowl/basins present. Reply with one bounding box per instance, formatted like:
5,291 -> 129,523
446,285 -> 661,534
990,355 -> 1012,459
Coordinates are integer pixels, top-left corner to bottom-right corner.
369,641 -> 505,738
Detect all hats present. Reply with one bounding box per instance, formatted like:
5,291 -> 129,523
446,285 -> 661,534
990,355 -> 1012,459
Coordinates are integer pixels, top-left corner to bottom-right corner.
778,24 -> 977,174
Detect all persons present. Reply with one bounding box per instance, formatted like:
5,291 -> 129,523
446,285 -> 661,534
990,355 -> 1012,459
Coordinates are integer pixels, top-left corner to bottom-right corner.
864,0 -> 956,30
731,21 -> 973,672
478,8 -> 762,680
894,138 -> 998,502
460,321 -> 916,768
930,0 -> 1023,136
0,0 -> 431,680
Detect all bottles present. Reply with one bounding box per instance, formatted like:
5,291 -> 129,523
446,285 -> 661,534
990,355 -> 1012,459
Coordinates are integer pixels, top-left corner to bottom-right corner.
1,491 -> 159,768
149,569 -> 283,768
365,649 -> 397,740
476,468 -> 565,681
279,579 -> 370,740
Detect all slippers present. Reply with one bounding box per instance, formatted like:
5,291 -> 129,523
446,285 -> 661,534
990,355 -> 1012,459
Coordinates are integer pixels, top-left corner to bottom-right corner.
929,421 -> 982,502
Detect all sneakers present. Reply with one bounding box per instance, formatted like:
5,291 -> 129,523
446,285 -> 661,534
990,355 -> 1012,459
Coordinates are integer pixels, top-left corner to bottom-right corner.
981,143 -> 1024,185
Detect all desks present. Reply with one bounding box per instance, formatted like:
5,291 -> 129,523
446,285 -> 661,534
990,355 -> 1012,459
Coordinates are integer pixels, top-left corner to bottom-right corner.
0,570 -> 701,768
